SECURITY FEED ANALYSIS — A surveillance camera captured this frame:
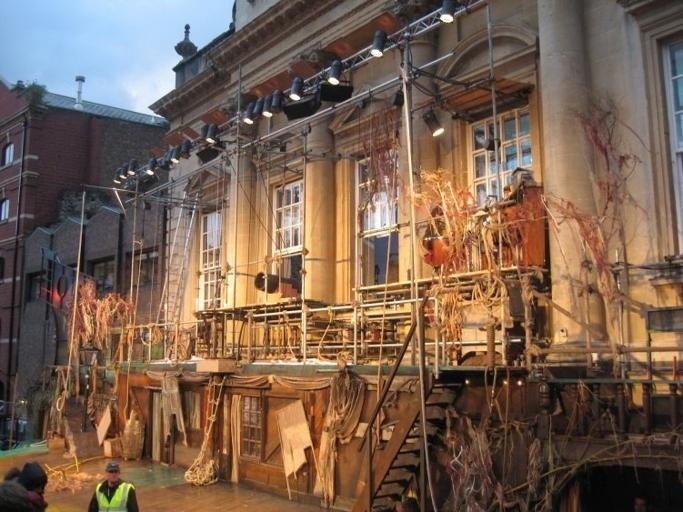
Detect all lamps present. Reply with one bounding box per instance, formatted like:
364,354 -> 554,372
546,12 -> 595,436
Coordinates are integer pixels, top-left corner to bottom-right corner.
201,123 -> 217,144
371,30 -> 386,58
328,60 -> 342,85
289,77 -> 304,101
114,159 -> 137,184
440,1 -> 455,23
243,88 -> 282,124
146,158 -> 157,175
422,109 -> 444,137
158,139 -> 190,171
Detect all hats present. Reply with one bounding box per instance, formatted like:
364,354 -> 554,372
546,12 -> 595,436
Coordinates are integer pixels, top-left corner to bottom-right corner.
104,460 -> 121,473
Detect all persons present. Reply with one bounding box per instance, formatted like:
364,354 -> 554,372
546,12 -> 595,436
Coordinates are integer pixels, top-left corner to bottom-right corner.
1,462 -> 48,512
85,461 -> 139,512
